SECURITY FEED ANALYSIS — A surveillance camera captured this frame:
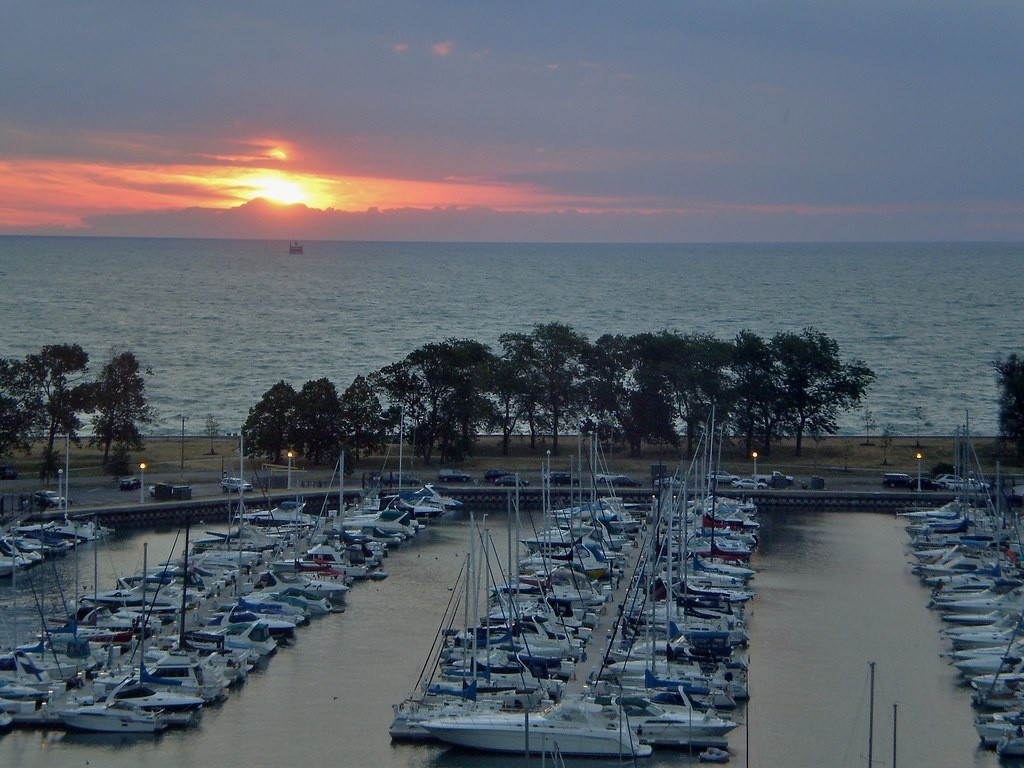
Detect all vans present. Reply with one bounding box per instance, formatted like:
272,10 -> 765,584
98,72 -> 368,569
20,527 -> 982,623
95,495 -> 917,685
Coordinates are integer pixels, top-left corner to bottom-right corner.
882,472 -> 914,487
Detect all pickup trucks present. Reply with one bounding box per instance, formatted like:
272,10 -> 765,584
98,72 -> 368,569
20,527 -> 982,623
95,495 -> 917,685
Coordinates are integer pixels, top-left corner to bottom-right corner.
551,474 -> 579,486
752,471 -> 794,484
381,472 -> 422,487
946,479 -> 991,492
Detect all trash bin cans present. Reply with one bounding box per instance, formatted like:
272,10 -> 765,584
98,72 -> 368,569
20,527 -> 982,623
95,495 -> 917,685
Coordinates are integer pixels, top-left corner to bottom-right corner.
811,474 -> 826,490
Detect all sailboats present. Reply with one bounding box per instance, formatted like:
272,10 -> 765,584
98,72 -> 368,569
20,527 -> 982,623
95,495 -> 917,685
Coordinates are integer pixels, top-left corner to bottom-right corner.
2,427 -> 1024,767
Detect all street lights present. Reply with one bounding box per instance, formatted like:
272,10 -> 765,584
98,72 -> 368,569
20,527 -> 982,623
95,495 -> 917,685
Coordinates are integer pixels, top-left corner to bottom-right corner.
176,414 -> 189,477
139,463 -> 146,501
916,454 -> 922,492
57,469 -> 63,510
752,452 -> 758,488
288,451 -> 293,491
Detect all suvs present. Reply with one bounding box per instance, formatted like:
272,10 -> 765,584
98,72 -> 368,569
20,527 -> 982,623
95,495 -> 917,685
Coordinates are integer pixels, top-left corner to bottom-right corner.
34,490 -> 65,508
221,477 -> 253,493
439,468 -> 471,482
705,470 -> 741,484
119,477 -> 142,490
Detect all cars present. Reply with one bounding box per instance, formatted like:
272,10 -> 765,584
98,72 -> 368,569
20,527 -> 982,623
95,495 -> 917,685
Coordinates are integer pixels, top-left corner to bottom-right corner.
542,471 -> 560,481
484,469 -> 518,481
907,477 -> 942,492
148,485 -> 177,497
934,474 -> 962,483
595,472 -> 626,482
731,480 -> 767,490
494,476 -> 530,487
655,478 -> 686,487
609,477 -> 644,488
